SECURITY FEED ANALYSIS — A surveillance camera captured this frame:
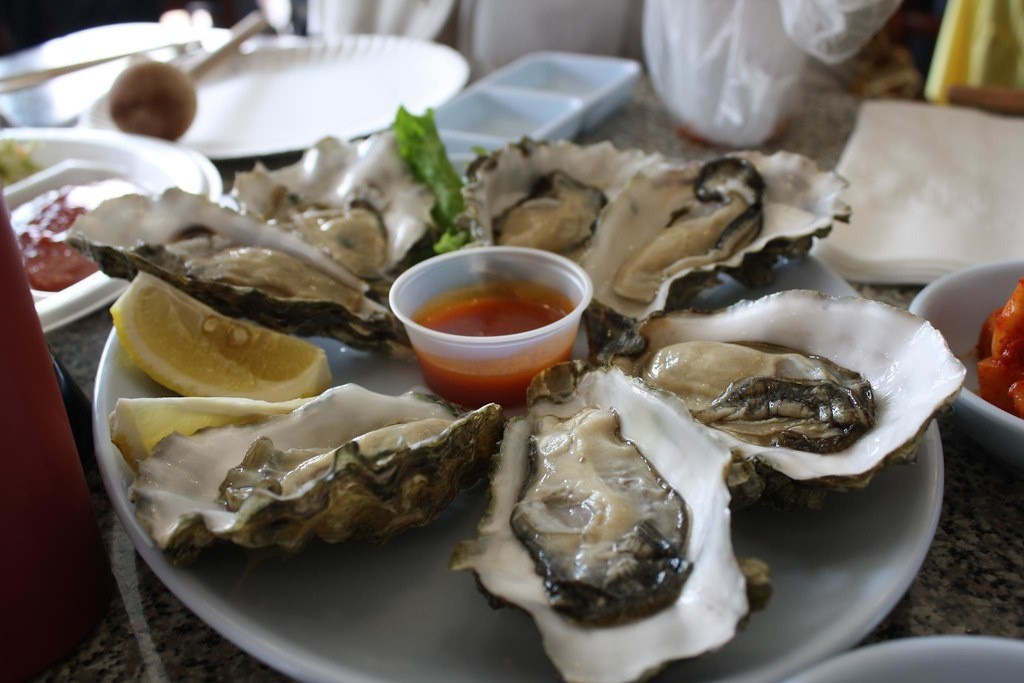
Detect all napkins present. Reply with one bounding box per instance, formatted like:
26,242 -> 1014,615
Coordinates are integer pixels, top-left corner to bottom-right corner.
811,100 -> 1024,283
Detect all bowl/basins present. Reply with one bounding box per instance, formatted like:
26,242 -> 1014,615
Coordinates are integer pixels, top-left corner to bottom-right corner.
782,633 -> 1024,683
388,245 -> 594,410
909,257 -> 1024,467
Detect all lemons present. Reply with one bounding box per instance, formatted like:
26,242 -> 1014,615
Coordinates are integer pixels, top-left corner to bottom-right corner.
108,397 -> 319,474
110,269 -> 331,401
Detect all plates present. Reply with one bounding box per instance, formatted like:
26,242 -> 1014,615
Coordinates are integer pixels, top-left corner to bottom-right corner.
92,36 -> 470,160
0,126 -> 223,335
92,257 -> 946,683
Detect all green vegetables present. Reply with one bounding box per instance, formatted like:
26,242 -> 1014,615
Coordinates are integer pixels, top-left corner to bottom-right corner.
393,105 -> 485,250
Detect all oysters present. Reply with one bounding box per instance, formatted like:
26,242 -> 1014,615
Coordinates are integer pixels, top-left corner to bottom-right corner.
69,137 -> 967,683
